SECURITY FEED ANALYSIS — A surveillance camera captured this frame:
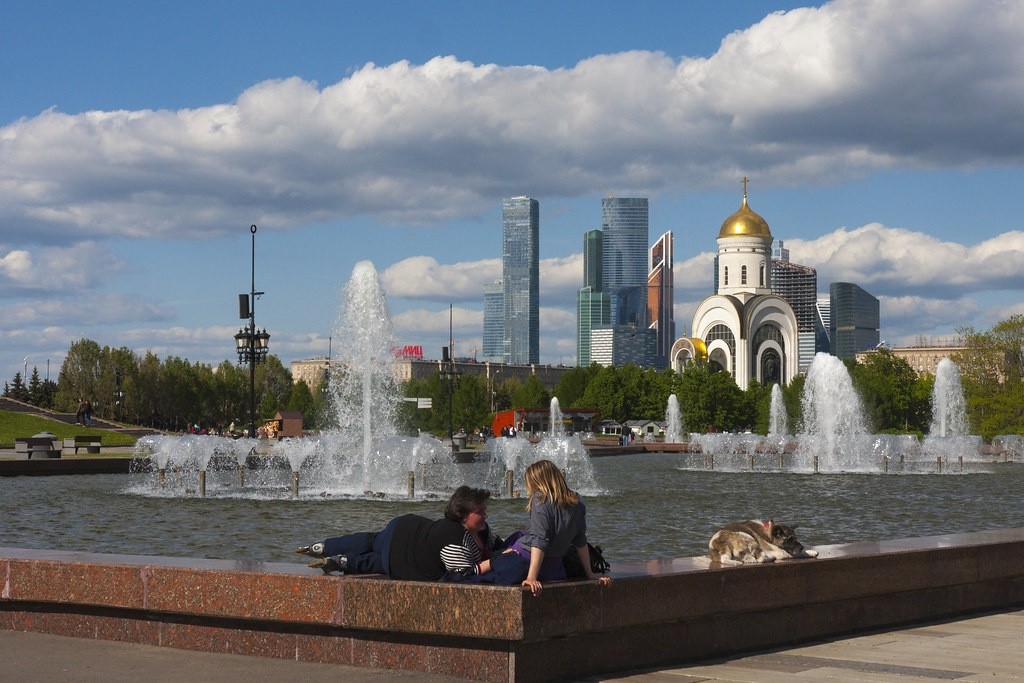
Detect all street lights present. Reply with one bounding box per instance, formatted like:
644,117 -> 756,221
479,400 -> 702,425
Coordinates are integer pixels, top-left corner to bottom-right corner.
235,327 -> 272,440
438,355 -> 463,444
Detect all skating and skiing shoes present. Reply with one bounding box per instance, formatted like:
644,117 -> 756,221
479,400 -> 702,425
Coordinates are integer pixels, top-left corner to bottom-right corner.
308,554 -> 348,574
294,541 -> 325,559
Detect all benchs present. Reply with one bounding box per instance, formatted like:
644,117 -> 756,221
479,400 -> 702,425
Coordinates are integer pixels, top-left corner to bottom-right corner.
15,437 -> 54,459
74,435 -> 102,454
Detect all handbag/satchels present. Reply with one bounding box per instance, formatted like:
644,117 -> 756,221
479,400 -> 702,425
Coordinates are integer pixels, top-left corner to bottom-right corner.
513,426 -> 516,437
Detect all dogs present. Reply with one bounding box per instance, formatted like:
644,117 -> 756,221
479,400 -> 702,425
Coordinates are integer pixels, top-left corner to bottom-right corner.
708,517 -> 820,567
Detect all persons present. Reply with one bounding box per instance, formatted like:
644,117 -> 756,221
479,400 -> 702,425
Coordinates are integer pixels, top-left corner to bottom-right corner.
179,422 -> 248,438
294,460 -> 615,597
75,398 -> 94,428
474,422 -> 635,447
